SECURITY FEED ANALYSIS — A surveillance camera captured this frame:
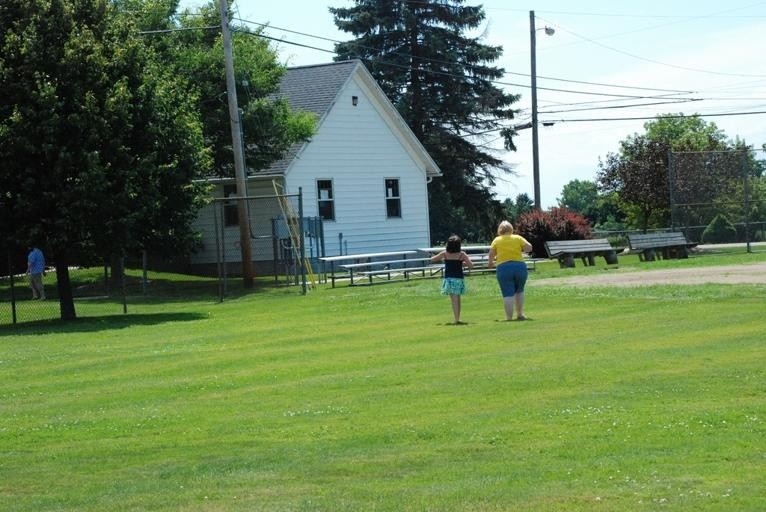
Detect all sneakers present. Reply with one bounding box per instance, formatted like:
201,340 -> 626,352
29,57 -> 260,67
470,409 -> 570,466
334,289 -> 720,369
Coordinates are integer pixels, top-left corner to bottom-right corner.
455,315 -> 533,326
30,296 -> 46,301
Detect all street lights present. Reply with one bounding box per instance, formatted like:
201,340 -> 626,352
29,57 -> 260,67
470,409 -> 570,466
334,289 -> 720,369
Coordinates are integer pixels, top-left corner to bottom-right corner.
529,8 -> 560,214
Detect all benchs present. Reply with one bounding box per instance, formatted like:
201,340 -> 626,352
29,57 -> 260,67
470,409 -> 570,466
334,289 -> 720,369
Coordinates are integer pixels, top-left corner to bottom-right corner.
317,244 -> 544,288
626,231 -> 699,261
543,238 -> 625,268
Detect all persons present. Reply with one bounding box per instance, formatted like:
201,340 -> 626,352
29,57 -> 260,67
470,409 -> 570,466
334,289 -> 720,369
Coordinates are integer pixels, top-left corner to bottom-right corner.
487,219 -> 534,325
25,244 -> 48,302
430,235 -> 474,324
